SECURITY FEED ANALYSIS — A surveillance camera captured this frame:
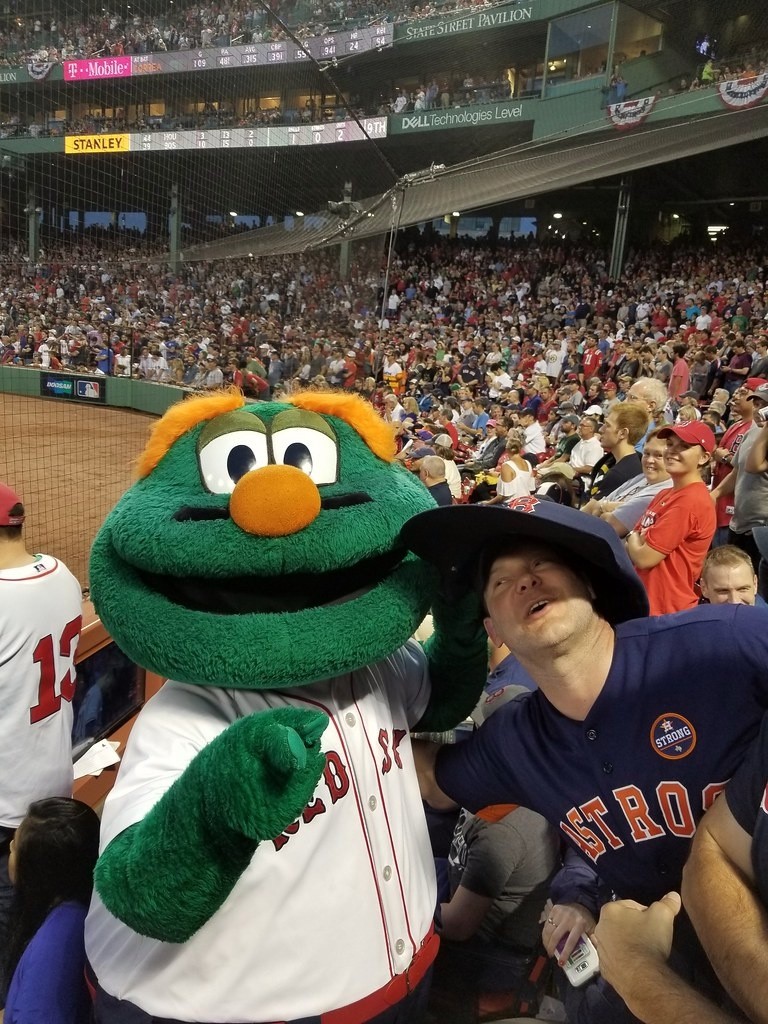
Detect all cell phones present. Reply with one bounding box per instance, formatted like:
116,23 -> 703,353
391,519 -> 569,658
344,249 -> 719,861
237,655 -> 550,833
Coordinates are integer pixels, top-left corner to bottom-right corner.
553,930 -> 599,987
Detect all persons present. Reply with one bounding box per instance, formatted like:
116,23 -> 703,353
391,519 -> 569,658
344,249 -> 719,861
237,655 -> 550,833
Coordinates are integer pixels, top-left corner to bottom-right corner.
411,541 -> 768,899
0,486 -> 84,929
0,220 -> 767,1024
0,0 -> 768,128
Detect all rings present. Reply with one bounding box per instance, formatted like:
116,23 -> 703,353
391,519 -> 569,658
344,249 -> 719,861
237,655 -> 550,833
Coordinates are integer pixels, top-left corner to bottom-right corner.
548,919 -> 557,927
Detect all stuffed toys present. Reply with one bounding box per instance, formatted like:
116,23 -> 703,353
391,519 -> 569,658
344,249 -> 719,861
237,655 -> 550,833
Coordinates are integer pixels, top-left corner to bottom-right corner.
82,388 -> 488,1024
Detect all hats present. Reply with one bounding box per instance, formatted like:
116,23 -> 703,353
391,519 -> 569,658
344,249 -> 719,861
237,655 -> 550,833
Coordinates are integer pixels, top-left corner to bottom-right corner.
551,339 -> 562,347
741,377 -> 768,405
443,396 -> 458,407
498,384 -> 510,392
486,419 -> 497,428
680,324 -> 687,329
0,483 -> 26,525
501,403 -> 519,410
679,390 -> 700,401
353,343 -> 360,349
404,430 -> 453,459
590,377 -> 601,384
556,400 -> 579,426
601,382 -> 617,390
450,383 -> 461,391
384,350 -> 395,356
519,407 -> 536,417
346,351 -> 356,358
619,376 -> 633,382
520,378 -> 536,392
657,420 -> 716,456
511,335 -> 521,342
399,494 -> 649,627
409,378 -> 419,388
332,340 -> 340,345
